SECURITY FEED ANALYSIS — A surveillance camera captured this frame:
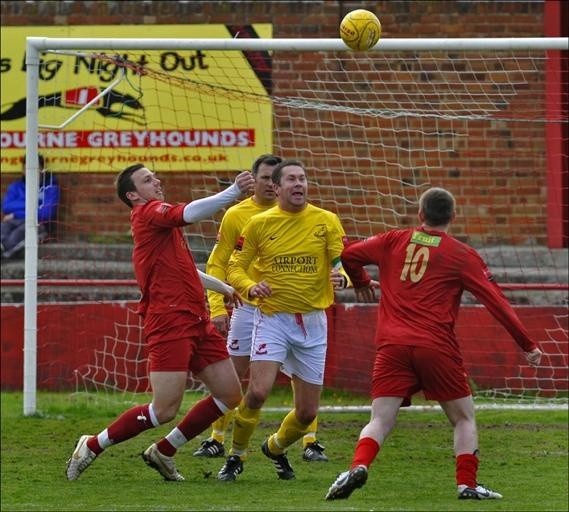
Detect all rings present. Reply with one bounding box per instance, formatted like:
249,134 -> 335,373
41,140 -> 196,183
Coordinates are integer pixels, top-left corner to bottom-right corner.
340,279 -> 342,287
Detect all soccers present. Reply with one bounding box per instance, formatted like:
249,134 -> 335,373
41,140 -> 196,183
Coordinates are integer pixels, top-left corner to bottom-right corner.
340,8 -> 382,50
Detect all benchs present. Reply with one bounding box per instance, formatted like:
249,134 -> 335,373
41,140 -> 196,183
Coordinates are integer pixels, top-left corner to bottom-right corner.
2,241 -> 569,311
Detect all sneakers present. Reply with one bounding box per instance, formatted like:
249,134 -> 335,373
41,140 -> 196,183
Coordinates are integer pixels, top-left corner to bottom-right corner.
326,465 -> 368,501
66,435 -> 97,481
457,484 -> 503,498
142,444 -> 185,481
194,438 -> 225,457
303,440 -> 329,463
218,454 -> 243,482
261,439 -> 295,479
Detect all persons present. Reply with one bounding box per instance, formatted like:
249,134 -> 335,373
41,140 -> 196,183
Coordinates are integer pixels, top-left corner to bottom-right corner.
325,187 -> 542,499
193,154 -> 330,461
0,154 -> 59,259
217,158 -> 352,481
66,163 -> 255,482
